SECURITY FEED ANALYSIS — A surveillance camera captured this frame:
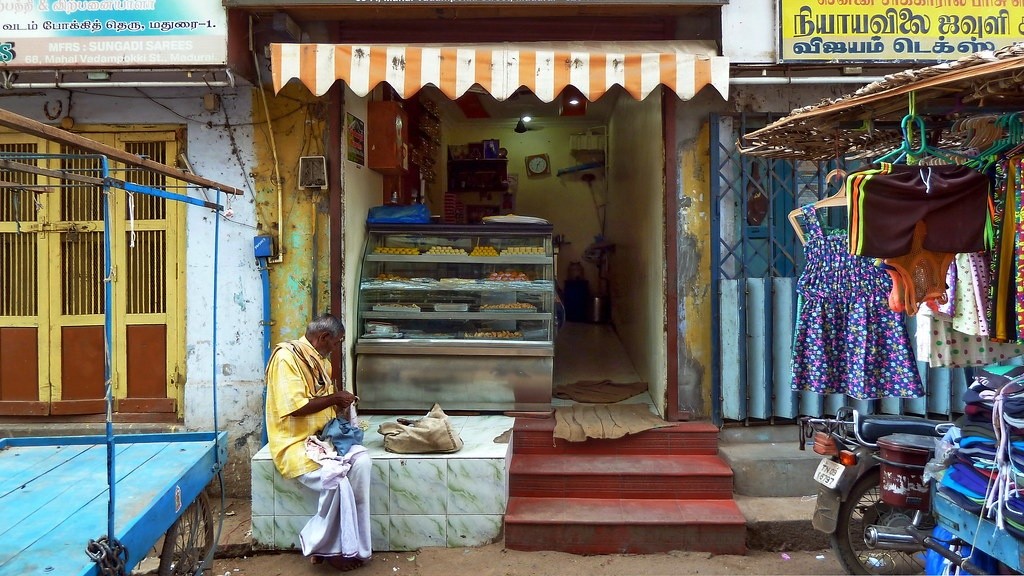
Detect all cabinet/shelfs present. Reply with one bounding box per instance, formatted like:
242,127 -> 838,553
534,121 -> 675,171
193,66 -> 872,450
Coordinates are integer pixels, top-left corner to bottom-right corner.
447,158 -> 509,193
354,223 -> 555,356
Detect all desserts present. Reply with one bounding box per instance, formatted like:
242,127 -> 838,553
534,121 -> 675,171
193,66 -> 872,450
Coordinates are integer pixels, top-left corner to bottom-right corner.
373,246 -> 544,338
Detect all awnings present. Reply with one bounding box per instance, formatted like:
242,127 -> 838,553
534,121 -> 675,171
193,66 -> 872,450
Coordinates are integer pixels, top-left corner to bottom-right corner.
269,38 -> 731,103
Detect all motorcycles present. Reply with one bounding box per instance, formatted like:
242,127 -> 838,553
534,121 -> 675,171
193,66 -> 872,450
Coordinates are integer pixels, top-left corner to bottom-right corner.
796,405 -> 956,576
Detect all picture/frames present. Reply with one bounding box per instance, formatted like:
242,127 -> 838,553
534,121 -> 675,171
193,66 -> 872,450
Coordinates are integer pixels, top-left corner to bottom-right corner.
483,139 -> 499,159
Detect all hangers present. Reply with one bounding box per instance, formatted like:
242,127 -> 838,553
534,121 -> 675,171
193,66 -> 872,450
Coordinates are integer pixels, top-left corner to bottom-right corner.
788,110 -> 1024,247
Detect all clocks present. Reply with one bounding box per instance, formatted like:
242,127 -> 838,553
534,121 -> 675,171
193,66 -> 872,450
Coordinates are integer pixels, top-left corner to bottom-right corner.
525,152 -> 550,177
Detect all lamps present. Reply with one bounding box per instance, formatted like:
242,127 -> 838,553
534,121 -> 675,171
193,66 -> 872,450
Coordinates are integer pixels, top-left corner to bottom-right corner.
514,117 -> 528,133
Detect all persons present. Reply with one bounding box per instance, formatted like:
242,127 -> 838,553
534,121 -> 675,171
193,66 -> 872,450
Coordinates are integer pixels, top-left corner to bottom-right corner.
485,140 -> 498,158
265,312 -> 373,572
